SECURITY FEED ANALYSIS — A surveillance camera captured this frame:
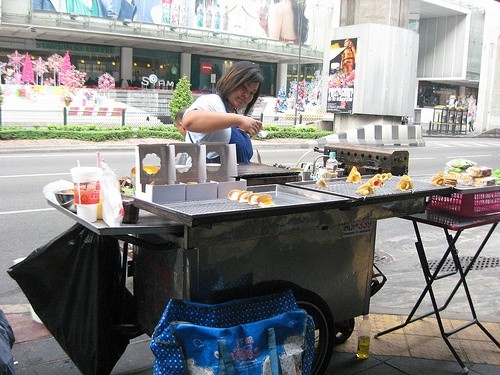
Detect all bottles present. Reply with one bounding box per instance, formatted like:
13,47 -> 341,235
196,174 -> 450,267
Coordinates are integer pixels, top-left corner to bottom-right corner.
326,151 -> 338,178
356,315 -> 370,359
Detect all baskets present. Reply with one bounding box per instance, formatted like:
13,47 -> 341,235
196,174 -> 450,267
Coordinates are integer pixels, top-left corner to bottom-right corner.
427,184 -> 500,216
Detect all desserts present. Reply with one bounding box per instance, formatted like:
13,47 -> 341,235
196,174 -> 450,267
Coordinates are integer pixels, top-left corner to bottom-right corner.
315,166 -> 414,195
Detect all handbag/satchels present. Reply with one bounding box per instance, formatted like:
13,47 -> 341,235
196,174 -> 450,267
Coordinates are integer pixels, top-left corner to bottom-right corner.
149,288 -> 317,373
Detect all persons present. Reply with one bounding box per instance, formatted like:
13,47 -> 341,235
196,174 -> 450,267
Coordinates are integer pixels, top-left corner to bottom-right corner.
259,0 -> 309,45
181,61 -> 265,163
175,108 -> 187,136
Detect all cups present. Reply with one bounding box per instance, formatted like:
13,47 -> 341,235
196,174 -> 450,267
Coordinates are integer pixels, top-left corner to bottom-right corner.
122,194 -> 139,224
71,166 -> 101,222
302,167 -> 345,181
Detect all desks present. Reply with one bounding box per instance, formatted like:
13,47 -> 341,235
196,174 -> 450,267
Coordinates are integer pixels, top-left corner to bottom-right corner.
373,209 -> 500,373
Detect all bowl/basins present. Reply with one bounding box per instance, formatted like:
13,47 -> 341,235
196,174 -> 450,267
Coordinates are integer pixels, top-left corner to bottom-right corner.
55,189 -> 77,213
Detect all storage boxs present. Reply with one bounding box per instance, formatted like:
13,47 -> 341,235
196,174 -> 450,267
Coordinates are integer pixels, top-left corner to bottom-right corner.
426,185 -> 500,217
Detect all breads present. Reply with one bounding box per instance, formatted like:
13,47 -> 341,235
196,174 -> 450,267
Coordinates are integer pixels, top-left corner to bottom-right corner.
459,165 -> 495,187
228,190 -> 273,208
